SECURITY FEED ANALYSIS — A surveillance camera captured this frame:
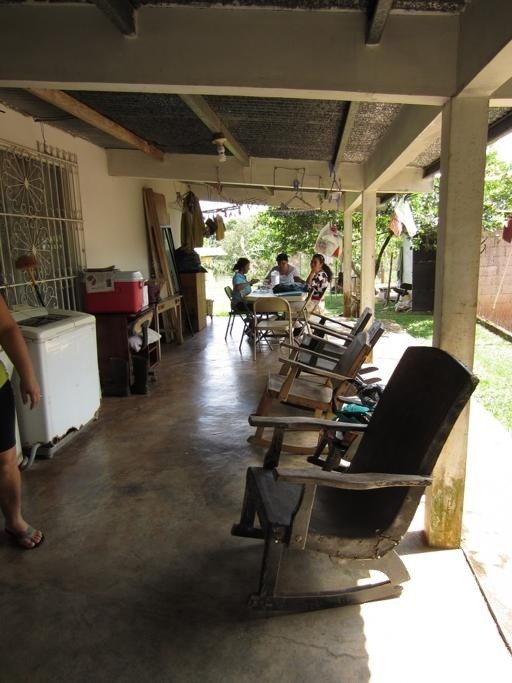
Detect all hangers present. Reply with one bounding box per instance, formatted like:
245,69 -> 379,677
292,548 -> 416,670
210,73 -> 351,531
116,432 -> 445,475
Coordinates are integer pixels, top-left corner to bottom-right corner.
277,186 -> 314,210
167,192 -> 183,211
330,170 -> 342,194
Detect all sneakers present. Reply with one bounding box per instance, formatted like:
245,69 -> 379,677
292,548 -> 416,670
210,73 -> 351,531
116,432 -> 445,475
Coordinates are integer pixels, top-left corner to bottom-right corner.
245,326 -> 305,342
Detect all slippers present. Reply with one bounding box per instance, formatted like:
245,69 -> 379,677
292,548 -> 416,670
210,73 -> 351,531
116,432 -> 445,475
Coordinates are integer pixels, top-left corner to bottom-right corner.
5,525 -> 45,549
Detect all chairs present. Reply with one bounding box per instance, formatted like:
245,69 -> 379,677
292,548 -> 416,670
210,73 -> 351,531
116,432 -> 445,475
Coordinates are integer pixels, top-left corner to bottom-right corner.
295,308 -> 371,358
230,346 -> 479,611
247,318 -> 386,454
222,285 -> 324,360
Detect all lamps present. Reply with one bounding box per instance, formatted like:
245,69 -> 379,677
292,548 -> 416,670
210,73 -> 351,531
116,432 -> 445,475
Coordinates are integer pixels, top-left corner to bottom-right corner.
212,131 -> 227,162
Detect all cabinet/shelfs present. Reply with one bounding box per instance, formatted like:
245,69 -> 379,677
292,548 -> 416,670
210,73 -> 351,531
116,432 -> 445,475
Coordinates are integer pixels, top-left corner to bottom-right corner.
180,269 -> 208,332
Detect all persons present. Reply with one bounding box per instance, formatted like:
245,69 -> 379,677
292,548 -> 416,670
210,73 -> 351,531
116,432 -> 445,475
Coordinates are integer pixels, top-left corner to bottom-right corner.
231,257 -> 263,341
263,252 -> 306,285
288,254 -> 334,337
1,287 -> 45,550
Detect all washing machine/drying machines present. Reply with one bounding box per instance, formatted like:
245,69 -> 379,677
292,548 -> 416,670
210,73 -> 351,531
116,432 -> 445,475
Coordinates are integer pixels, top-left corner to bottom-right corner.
7,303 -> 104,459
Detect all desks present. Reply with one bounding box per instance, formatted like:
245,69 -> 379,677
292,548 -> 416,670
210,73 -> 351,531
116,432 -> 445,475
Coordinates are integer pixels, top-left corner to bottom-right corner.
96,294 -> 186,396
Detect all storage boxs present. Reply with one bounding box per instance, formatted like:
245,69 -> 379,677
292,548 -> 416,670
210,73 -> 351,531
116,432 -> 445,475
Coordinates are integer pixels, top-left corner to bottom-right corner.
80,270 -> 143,314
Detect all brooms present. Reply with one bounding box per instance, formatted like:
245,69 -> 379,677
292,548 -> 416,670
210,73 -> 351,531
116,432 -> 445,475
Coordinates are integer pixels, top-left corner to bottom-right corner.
15,255 -> 46,308
